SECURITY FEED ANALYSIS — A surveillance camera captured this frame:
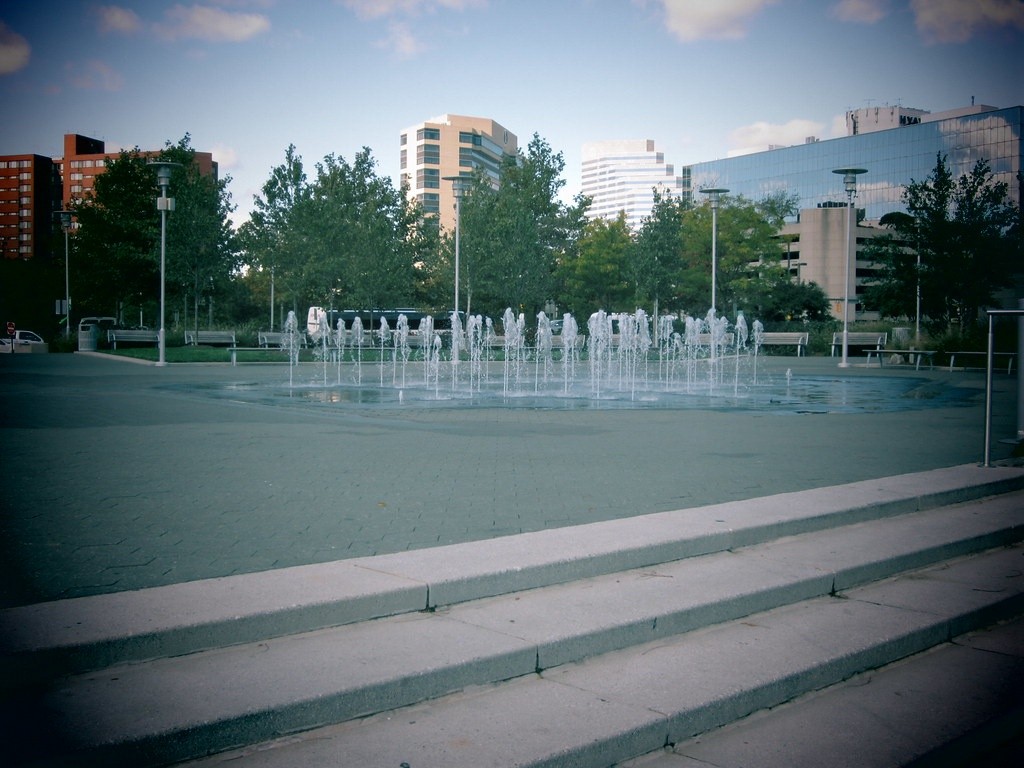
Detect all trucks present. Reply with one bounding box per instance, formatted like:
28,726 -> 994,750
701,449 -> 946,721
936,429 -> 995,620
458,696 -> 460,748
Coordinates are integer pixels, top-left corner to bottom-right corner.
0,330 -> 44,353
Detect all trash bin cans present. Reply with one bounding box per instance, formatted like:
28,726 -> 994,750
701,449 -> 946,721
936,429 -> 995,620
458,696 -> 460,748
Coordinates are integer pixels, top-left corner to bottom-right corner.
78,324 -> 97,352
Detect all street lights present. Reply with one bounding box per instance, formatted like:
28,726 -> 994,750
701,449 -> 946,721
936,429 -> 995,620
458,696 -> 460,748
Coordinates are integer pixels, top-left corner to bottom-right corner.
146,162 -> 183,367
699,188 -> 730,309
54,210 -> 72,340
441,175 -> 474,315
832,168 -> 868,368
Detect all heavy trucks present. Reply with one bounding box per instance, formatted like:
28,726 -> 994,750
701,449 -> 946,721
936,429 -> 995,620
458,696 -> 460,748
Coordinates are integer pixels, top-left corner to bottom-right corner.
307,307 -> 469,335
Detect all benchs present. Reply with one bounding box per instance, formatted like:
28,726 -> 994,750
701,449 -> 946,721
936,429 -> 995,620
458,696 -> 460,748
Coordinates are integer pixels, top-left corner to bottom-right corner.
587,334 -> 652,349
184,331 -> 239,348
828,332 -> 888,358
328,346 -> 399,362
680,333 -> 734,354
107,330 -> 160,350
538,335 -> 585,350
395,335 -> 436,346
752,332 -> 809,357
257,332 -> 311,349
863,350 -> 937,372
226,348 -> 302,365
482,335 -> 525,349
945,351 -> 1018,374
332,335 -> 372,346
